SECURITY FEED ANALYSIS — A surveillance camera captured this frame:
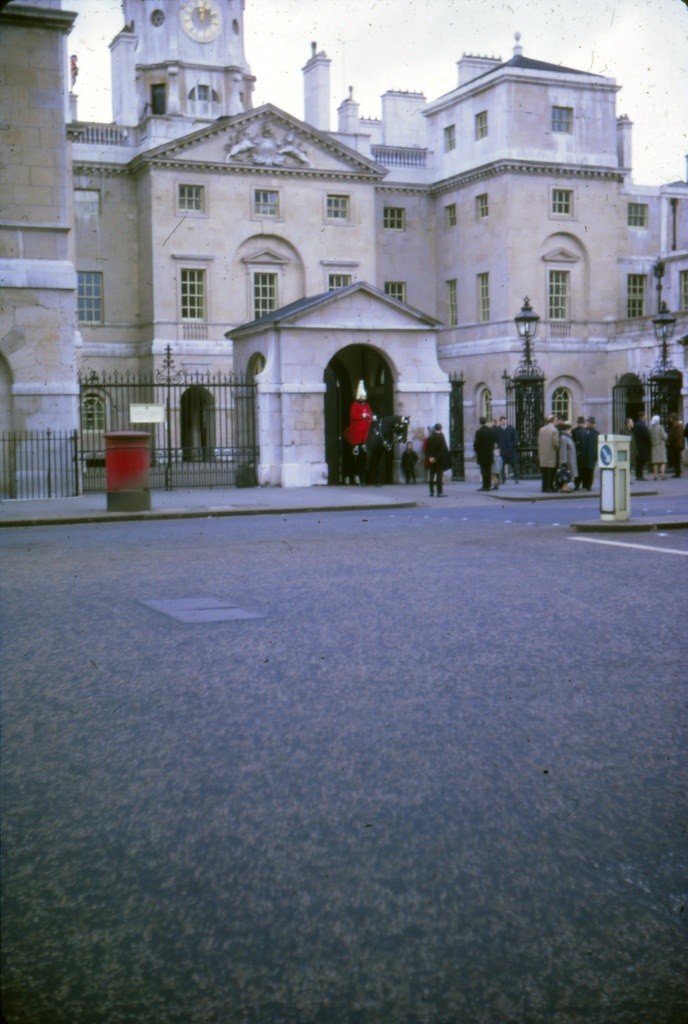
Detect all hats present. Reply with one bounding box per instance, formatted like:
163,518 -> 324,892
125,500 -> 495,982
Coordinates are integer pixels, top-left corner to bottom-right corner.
577,416 -> 585,424
356,380 -> 366,400
586,417 -> 595,424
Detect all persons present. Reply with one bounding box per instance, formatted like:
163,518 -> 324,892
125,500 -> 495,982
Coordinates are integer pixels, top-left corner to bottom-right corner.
398,442 -> 420,484
489,441 -> 504,490
347,379 -> 379,454
577,417 -> 599,491
633,410 -> 652,481
666,412 -> 685,479
490,418 -> 503,448
620,417 -> 638,485
422,423 -> 453,498
537,415 -> 560,493
560,422 -> 579,493
647,414 -> 668,481
473,418 -> 493,492
493,416 -> 520,484
570,416 -> 585,457
556,416 -> 567,428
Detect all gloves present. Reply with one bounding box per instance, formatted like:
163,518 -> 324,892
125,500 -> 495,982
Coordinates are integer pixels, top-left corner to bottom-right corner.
372,415 -> 377,420
363,413 -> 367,418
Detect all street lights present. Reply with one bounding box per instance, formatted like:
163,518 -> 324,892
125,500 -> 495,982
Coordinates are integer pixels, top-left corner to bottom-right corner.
514,296 -> 542,376
652,300 -> 677,373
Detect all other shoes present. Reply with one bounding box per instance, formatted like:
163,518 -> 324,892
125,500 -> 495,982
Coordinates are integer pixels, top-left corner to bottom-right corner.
353,445 -> 359,454
586,487 -> 591,491
562,483 -> 575,492
636,477 -> 646,480
438,494 -> 448,497
573,487 -> 579,490
477,486 -> 492,491
430,490 -> 434,496
672,474 -> 681,478
542,487 -> 557,492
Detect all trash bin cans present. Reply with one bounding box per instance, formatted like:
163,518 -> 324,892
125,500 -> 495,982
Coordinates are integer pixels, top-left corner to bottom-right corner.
597,433 -> 632,521
103,431 -> 153,511
